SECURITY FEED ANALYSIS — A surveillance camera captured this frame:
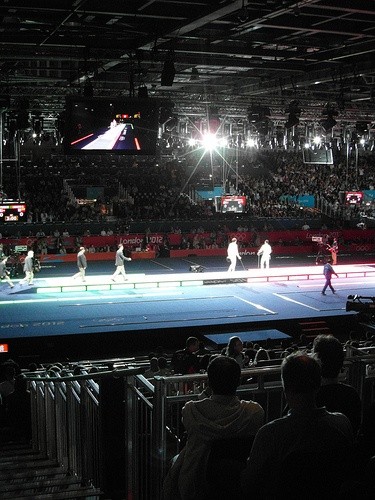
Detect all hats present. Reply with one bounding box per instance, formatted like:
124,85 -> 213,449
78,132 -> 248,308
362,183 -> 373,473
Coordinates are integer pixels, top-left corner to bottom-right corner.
328,259 -> 333,262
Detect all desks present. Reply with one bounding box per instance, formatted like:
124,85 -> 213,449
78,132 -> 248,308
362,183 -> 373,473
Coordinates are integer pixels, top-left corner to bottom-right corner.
201,328 -> 292,349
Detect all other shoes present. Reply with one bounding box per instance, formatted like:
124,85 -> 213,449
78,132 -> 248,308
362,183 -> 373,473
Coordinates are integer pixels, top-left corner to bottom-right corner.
321,292 -> 326,296
333,290 -> 336,294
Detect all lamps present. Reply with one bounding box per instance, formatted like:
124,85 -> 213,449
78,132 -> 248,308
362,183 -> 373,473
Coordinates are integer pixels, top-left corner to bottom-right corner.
5,106 -> 47,145
247,105 -> 272,137
138,81 -> 148,98
285,110 -> 300,129
160,56 -> 175,87
356,121 -> 372,134
169,125 -> 374,154
319,107 -> 340,132
84,78 -> 94,100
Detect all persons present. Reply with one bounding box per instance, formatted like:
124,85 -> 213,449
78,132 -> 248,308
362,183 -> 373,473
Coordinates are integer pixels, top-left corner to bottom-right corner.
0,227 -> 70,255
167,221 -> 343,234
144,233 -> 174,257
313,334 -> 362,431
238,350 -> 353,500
315,239 -> 338,265
179,234 -> 344,251
163,356 -> 265,500
321,259 -> 338,295
71,247 -> 87,282
150,330 -> 375,383
227,238 -> 242,273
257,239 -> 271,269
0,148 -> 375,223
110,244 -> 132,282
0,358 -> 113,441
0,250 -> 35,287
73,228 -> 120,252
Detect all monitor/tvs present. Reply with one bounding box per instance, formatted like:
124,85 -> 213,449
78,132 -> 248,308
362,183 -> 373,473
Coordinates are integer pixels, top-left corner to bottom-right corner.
63,96 -> 157,156
213,195 -> 247,213
0,203 -> 27,224
303,146 -> 333,166
